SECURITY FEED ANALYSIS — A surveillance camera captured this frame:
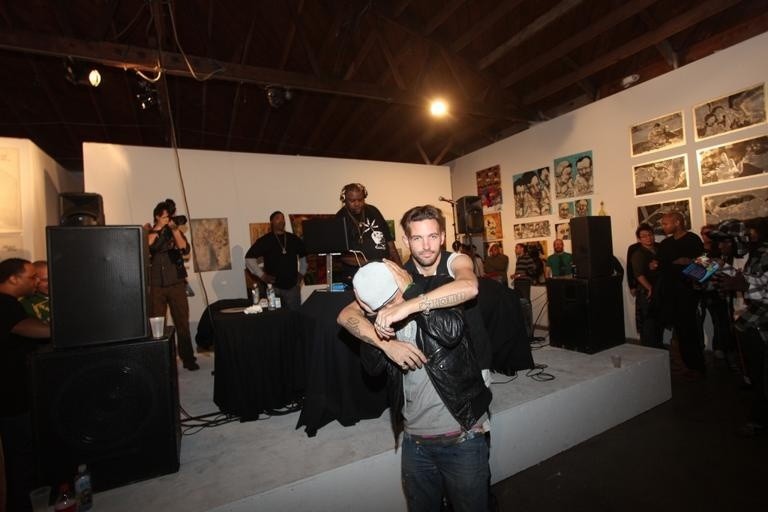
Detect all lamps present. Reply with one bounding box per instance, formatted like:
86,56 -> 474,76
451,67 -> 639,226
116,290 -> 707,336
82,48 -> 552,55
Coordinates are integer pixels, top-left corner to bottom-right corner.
66,66 -> 101,88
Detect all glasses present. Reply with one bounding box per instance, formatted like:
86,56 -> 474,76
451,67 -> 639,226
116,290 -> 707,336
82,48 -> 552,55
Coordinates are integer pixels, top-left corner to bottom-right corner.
19,273 -> 39,280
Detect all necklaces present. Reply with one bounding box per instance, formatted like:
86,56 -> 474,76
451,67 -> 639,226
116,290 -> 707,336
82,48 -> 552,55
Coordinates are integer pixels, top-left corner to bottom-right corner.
275,234 -> 290,256
344,207 -> 369,244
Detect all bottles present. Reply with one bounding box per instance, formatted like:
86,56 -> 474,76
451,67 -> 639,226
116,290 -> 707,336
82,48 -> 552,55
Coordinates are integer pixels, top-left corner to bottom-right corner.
55,484 -> 76,512
267,283 -> 276,311
75,464 -> 93,511
252,283 -> 259,305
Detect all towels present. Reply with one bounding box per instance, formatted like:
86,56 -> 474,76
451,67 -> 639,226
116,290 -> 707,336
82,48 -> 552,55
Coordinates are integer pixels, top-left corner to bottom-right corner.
244,305 -> 262,314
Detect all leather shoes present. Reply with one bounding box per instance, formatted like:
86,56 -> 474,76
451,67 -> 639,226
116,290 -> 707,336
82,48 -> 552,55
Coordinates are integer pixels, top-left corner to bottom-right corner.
183,360 -> 199,370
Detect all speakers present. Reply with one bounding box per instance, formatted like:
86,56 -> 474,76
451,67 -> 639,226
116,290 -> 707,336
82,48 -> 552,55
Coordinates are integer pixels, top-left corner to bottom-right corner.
545,277 -> 627,355
456,196 -> 484,234
570,216 -> 614,279
58,193 -> 105,226
46,226 -> 150,351
32,328 -> 180,506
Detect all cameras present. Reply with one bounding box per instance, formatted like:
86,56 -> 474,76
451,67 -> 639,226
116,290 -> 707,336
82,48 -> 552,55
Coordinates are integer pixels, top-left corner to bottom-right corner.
169,215 -> 187,224
166,248 -> 187,279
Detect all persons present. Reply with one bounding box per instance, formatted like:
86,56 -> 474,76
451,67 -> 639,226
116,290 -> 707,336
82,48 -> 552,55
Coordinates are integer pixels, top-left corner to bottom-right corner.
555,161 -> 575,199
538,166 -> 551,197
647,123 -> 665,146
702,142 -> 768,184
465,245 -> 486,276
353,258 -> 493,512
513,178 -> 530,218
336,205 -> 501,512
663,124 -> 680,143
510,243 -> 537,305
575,198 -> 588,216
704,114 -> 730,136
332,185 -> 404,280
557,223 -> 570,240
734,219 -> 768,431
712,106 -> 739,129
698,216 -> 756,383
653,212 -> 714,381
16,259 -> 51,325
544,238 -> 574,279
627,223 -> 668,345
514,221 -> 549,239
141,204 -> 198,375
558,203 -> 573,220
627,236 -> 645,344
523,171 -> 551,217
245,210 -> 311,310
483,245 -> 508,284
574,156 -> 593,196
695,223 -> 732,361
0,257 -> 54,350
452,241 -> 464,254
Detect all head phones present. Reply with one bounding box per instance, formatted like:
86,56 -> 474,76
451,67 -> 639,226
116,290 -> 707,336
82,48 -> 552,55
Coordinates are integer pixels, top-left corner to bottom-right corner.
339,183 -> 368,202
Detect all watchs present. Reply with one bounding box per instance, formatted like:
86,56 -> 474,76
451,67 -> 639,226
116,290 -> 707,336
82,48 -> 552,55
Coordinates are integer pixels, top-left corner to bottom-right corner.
418,295 -> 429,315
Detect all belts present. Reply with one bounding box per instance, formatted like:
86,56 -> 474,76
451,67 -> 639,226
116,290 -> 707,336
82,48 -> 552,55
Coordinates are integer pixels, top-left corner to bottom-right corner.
411,433 -> 476,446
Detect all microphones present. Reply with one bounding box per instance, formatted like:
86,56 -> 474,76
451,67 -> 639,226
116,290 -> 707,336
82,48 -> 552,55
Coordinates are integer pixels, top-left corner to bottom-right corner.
439,196 -> 454,207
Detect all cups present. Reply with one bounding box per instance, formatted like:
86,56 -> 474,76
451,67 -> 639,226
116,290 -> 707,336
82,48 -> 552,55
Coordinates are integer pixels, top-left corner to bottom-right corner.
150,316 -> 165,339
30,486 -> 50,512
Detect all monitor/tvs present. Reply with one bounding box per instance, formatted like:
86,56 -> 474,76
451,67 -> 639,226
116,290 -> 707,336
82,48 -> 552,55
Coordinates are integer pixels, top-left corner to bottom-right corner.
302,217 -> 352,255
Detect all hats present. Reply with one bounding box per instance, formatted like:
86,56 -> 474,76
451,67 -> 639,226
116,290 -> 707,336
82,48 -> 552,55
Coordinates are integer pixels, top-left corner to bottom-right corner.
352,262 -> 400,311
